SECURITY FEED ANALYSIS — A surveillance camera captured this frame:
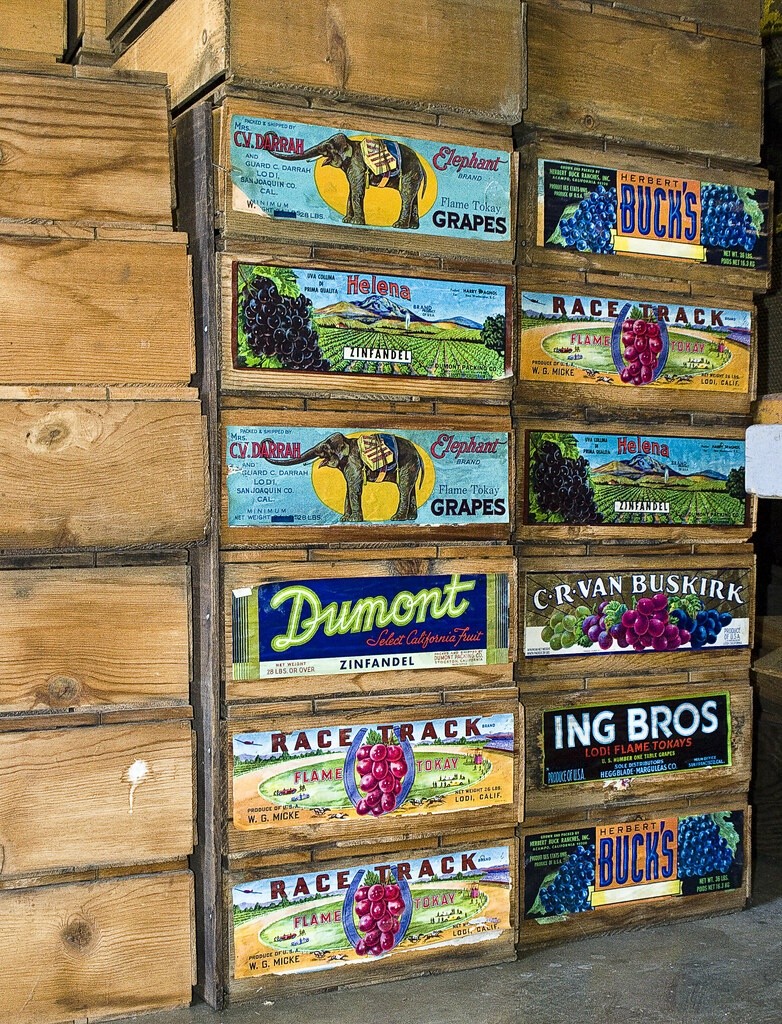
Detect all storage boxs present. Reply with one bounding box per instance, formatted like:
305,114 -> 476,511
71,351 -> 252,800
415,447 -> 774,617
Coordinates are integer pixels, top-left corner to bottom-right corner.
0,0 -> 775,1024
